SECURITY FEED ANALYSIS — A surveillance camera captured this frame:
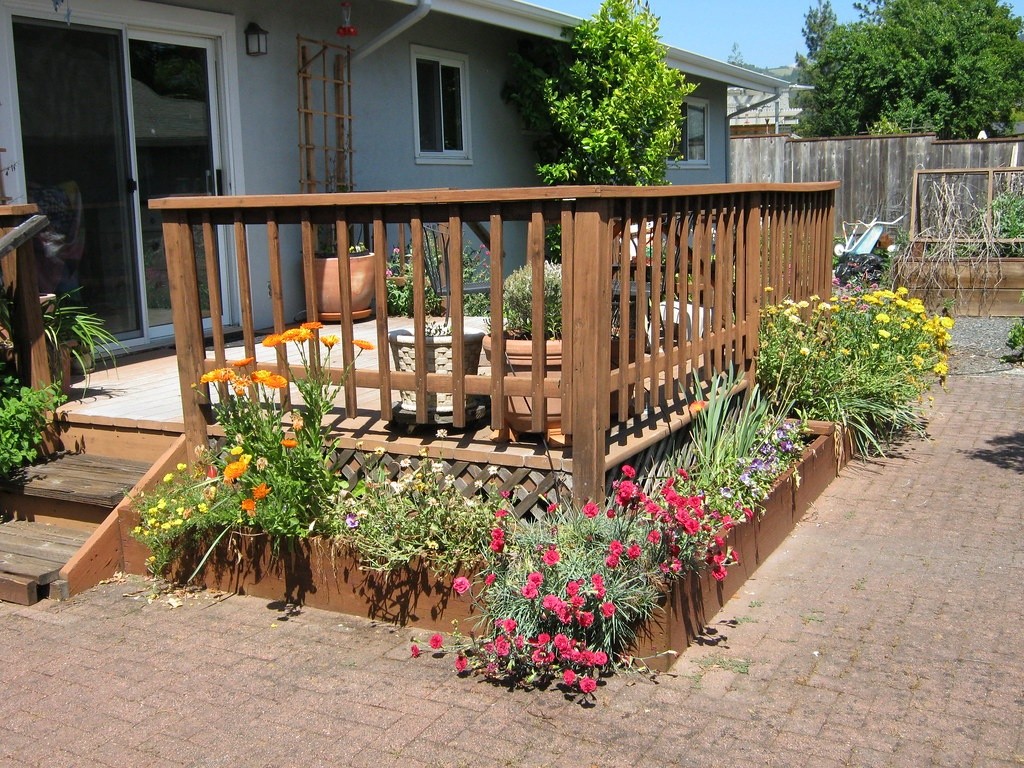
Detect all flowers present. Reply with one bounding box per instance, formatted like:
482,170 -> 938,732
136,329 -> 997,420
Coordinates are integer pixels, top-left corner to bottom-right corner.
349,241 -> 368,256
145,316 -> 807,691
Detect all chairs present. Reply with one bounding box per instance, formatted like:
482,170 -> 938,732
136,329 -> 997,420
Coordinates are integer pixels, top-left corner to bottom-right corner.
408,212 -> 694,350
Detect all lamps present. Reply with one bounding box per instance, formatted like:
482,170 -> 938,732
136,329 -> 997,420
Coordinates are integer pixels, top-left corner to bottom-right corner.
243,22 -> 269,56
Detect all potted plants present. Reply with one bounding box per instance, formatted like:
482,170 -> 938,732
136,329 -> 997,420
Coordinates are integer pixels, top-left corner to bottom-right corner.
387,325 -> 485,412
482,257 -> 565,430
0,279 -> 131,397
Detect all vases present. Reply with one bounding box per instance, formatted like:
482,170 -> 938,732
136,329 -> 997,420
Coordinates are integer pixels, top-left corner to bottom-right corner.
165,420 -> 852,671
301,255 -> 374,320
